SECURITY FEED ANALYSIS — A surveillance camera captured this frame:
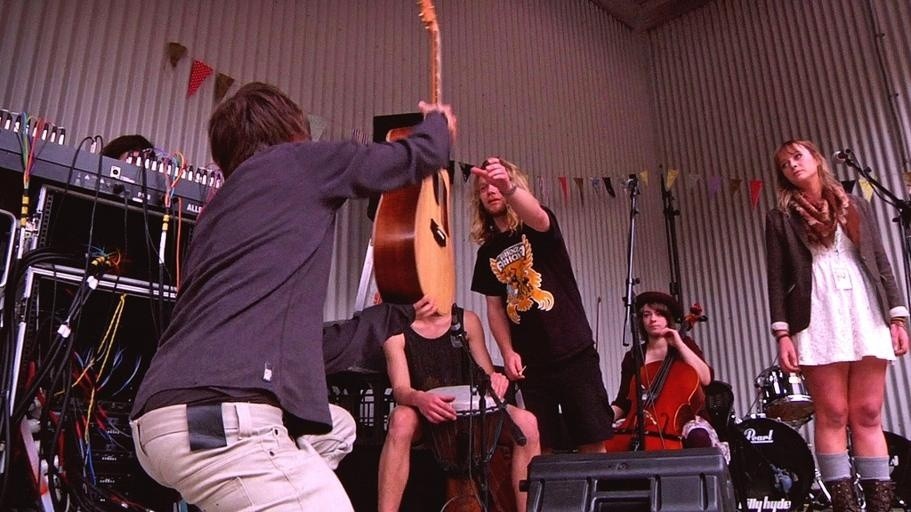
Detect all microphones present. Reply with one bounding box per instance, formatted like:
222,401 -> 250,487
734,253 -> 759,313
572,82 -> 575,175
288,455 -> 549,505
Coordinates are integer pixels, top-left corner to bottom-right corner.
771,463 -> 792,490
450,303 -> 462,336
625,178 -> 641,196
831,148 -> 851,164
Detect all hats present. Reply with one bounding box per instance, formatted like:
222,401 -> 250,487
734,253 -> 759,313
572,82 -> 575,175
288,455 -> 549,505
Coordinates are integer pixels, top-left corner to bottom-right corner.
635,290 -> 682,318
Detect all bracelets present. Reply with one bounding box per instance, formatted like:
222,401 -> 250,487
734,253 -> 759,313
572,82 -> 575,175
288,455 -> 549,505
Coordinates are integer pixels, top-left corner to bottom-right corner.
776,333 -> 790,342
891,315 -> 907,327
500,185 -> 517,196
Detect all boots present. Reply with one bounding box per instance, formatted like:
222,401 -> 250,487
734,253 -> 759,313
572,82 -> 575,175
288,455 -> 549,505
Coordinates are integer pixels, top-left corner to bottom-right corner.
828,476 -> 895,512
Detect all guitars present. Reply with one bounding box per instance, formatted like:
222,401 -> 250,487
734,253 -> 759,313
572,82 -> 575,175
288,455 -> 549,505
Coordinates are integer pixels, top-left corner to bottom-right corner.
373,2 -> 457,316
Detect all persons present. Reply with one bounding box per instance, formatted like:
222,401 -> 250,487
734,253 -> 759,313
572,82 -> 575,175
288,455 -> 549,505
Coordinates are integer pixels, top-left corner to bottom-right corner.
765,139 -> 910,510
99,134 -> 158,167
610,292 -> 718,429
129,80 -> 459,512
468,156 -> 614,454
377,303 -> 544,512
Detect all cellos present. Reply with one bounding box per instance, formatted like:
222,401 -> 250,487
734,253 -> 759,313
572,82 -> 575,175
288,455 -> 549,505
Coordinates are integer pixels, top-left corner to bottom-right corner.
605,301 -> 709,451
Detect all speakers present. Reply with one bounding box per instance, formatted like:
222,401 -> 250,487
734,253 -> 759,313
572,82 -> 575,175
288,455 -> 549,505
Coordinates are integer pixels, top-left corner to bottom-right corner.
366,111 -> 424,222
520,446 -> 738,512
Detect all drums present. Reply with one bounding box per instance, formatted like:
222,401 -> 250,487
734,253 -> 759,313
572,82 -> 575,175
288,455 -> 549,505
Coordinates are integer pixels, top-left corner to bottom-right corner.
423,384 -> 500,510
728,417 -> 816,512
754,364 -> 815,422
